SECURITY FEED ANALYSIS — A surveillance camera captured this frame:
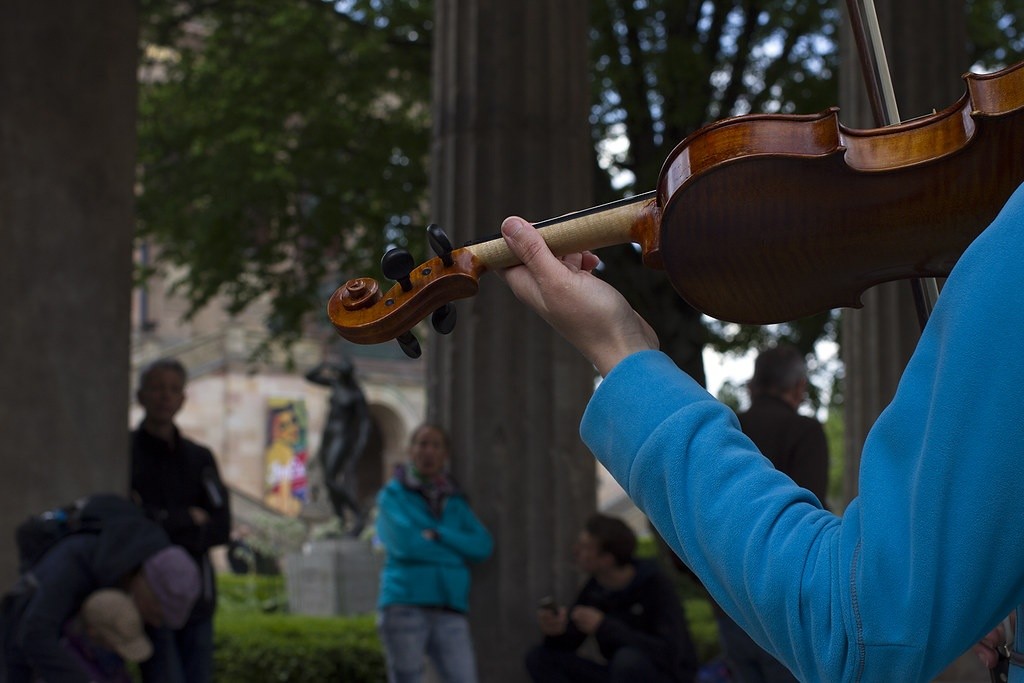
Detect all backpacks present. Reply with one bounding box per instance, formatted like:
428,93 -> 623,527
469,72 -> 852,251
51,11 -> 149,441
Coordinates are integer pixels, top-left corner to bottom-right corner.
16,494 -> 133,571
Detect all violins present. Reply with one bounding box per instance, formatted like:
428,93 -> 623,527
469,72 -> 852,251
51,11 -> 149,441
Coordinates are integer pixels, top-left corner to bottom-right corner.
327,63 -> 1024,359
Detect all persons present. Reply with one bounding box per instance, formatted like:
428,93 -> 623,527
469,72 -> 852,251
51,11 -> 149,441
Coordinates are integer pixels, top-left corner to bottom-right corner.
304,360 -> 383,540
0,358 -> 230,683
523,516 -> 698,683
500,183 -> 1024,683
701,347 -> 828,683
371,424 -> 496,683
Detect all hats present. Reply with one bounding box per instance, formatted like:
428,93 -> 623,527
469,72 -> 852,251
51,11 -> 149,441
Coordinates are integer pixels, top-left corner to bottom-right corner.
84,590 -> 154,666
133,546 -> 201,632
